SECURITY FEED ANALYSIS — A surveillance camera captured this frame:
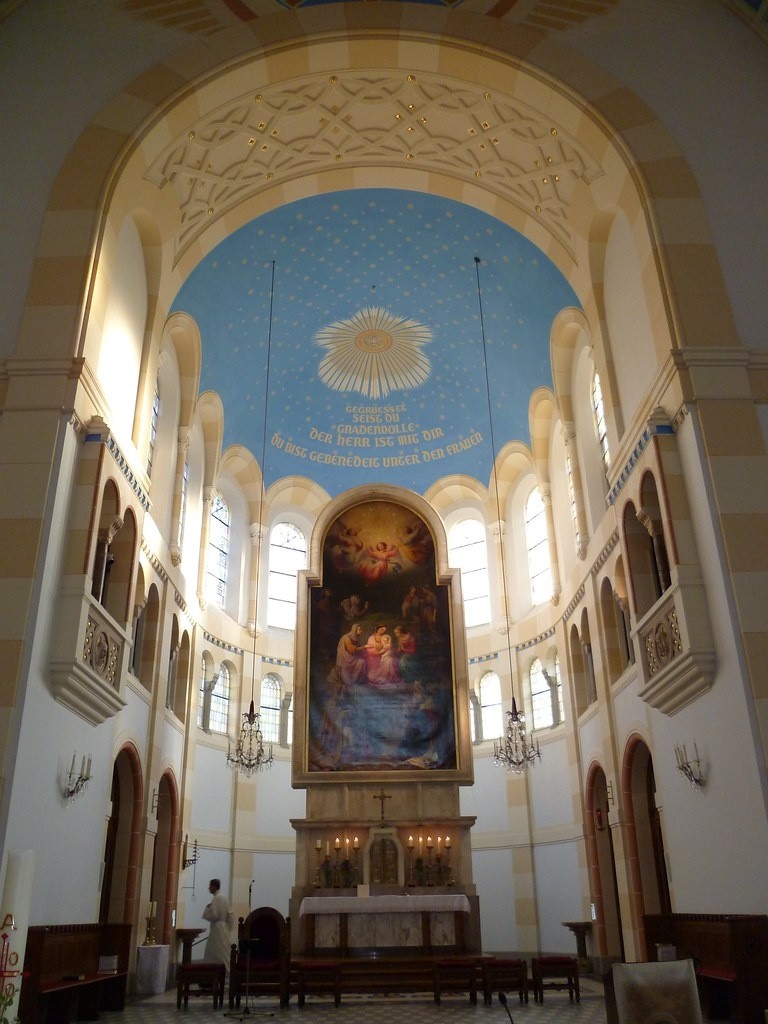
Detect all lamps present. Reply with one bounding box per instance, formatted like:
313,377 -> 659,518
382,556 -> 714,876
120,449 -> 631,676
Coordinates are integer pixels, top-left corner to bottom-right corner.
493,697 -> 541,776
227,700 -> 274,780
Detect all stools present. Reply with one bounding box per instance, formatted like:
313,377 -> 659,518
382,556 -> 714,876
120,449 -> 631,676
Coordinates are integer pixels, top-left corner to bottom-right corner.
532,956 -> 579,1003
175,962 -> 226,1010
483,959 -> 529,1005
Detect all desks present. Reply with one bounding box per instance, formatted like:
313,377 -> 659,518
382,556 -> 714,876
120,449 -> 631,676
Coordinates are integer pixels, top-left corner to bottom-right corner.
38,969 -> 128,1024
694,963 -> 738,1024
298,894 -> 470,955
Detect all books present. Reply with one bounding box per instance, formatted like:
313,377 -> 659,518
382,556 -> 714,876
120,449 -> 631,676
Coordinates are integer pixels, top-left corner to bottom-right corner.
64,975 -> 85,980
655,943 -> 676,961
97,955 -> 119,974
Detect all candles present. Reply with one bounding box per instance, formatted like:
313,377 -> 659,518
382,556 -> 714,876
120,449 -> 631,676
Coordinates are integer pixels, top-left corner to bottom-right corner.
346,840 -> 349,860
353,840 -> 359,848
418,839 -> 422,859
326,841 -> 329,856
408,838 -> 413,846
427,838 -> 432,846
674,742 -> 699,764
446,839 -> 450,846
152,902 -> 157,917
316,839 -> 321,848
335,840 -> 341,848
437,838 -> 440,854
68,750 -> 93,779
145,902 -> 153,918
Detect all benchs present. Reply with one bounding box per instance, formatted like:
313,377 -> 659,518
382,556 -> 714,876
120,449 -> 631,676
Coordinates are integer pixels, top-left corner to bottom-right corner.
288,953 -> 495,1007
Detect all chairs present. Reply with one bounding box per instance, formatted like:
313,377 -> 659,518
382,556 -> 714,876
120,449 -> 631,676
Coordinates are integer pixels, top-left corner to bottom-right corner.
604,958 -> 703,1024
229,907 -> 291,1010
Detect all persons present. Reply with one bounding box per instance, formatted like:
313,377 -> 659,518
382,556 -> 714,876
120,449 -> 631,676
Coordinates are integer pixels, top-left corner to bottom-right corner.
201,879 -> 234,976
